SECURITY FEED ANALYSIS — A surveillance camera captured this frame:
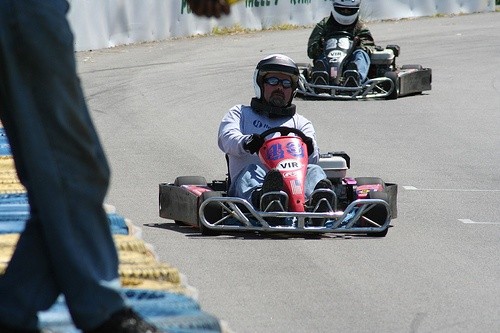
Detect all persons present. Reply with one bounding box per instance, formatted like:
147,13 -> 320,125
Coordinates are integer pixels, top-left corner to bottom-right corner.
308,0 -> 374,96
0,0 -> 158,333
218,54 -> 333,227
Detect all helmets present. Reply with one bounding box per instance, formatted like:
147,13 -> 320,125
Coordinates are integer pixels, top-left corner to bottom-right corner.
253,54 -> 300,99
331,0 -> 361,25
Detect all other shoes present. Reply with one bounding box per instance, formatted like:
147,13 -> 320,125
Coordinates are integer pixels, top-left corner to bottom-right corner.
314,60 -> 327,94
252,169 -> 283,226
310,179 -> 335,226
81,306 -> 160,333
345,61 -> 359,93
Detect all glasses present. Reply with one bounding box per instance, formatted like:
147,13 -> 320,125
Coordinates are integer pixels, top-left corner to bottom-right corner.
263,77 -> 292,88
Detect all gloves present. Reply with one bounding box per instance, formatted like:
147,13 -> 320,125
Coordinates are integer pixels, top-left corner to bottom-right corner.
243,133 -> 264,155
302,137 -> 313,157
354,36 -> 361,48
